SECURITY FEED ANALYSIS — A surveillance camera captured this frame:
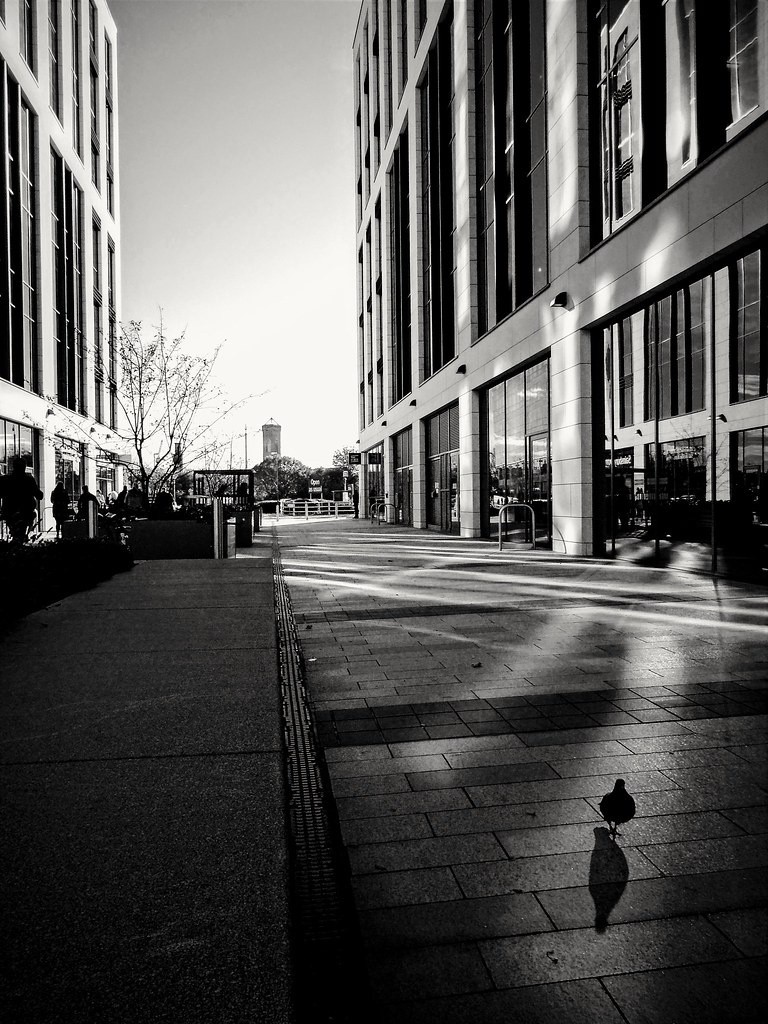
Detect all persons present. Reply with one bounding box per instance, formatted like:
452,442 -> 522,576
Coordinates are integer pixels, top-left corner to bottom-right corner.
352,490 -> 359,519
50,481 -> 191,539
0,455 -> 43,546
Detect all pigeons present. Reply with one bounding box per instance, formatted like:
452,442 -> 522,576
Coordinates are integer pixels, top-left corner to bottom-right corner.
597,779 -> 636,837
